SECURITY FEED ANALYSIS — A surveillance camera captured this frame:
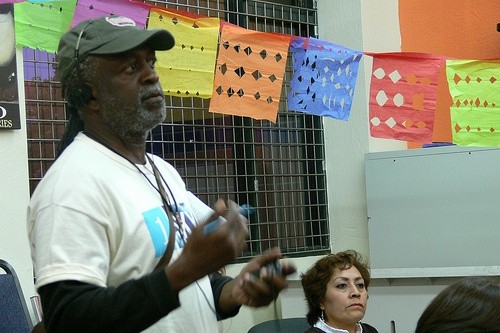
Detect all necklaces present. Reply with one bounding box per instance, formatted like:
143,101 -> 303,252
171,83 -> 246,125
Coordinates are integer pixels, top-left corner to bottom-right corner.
82,131 -> 184,241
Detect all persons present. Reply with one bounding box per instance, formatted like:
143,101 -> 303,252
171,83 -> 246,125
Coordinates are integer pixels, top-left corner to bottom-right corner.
299,249 -> 378,333
26,15 -> 297,333
415,277 -> 500,333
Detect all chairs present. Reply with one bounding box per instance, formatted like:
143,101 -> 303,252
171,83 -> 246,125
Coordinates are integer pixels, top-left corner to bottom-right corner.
0,259 -> 34,333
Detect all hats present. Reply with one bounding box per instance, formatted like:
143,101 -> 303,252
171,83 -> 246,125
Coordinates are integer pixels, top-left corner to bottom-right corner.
53,12 -> 176,98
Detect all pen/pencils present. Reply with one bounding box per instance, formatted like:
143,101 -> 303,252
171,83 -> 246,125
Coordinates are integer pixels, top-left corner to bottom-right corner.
204,205 -> 257,234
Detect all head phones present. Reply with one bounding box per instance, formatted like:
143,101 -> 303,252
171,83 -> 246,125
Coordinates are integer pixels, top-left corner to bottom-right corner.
67,19 -> 97,106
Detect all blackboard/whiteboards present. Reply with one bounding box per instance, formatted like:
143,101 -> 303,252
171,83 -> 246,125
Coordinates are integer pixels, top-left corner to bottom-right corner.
364,144 -> 500,280
